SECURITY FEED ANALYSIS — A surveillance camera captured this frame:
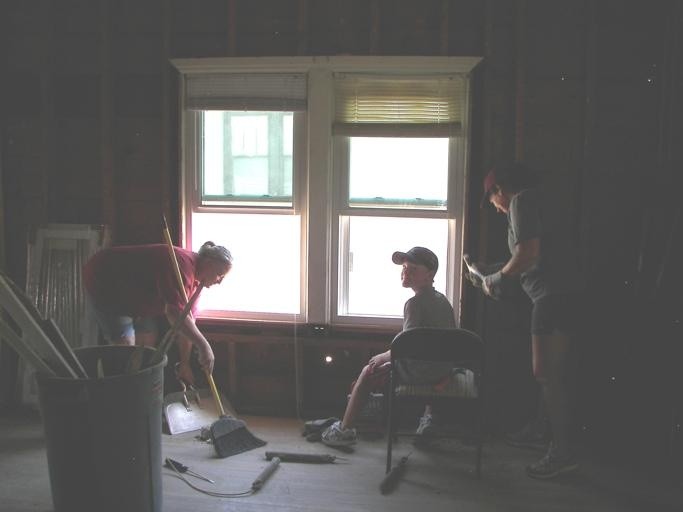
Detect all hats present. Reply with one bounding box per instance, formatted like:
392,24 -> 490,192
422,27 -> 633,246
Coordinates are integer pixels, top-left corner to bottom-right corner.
481,168 -> 518,208
393,247 -> 438,272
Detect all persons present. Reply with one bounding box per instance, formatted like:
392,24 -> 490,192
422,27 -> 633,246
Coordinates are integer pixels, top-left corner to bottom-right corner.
80,241 -> 230,386
318,246 -> 455,447
461,158 -> 605,479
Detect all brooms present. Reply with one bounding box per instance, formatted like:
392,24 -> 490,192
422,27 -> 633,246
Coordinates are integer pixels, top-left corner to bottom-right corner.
157,211 -> 269,458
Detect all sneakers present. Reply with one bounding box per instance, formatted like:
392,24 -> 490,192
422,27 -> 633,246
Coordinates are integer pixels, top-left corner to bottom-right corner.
321,425 -> 357,447
504,424 -> 547,449
526,454 -> 578,480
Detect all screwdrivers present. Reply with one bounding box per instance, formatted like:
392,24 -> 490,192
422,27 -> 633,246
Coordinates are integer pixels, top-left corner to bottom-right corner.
165,458 -> 216,484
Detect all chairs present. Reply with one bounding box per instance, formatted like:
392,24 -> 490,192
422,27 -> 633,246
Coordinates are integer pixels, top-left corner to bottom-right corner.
386,327 -> 483,483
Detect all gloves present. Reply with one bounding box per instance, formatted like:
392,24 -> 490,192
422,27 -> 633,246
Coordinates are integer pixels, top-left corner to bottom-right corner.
465,270 -> 491,289
480,271 -> 506,297
